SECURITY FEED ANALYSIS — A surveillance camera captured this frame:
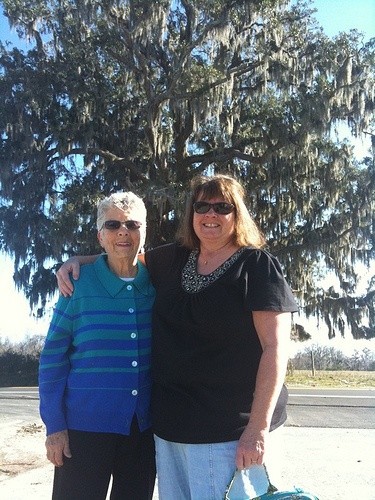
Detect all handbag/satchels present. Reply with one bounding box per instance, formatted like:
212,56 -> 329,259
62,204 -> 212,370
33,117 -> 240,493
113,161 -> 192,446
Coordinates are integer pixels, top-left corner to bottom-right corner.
225,462 -> 319,500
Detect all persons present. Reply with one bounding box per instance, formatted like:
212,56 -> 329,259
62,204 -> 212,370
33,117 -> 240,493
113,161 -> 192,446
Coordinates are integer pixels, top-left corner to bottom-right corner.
56,175 -> 299,500
38,190 -> 158,500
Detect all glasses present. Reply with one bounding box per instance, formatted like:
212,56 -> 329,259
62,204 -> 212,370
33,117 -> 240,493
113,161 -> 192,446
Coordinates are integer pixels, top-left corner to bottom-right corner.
99,220 -> 141,231
192,201 -> 235,215
